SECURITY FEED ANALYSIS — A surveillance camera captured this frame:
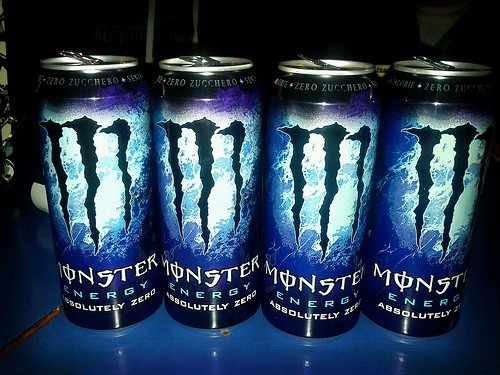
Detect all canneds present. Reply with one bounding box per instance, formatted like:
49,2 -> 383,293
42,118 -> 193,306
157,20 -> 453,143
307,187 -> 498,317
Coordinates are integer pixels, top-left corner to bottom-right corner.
263,53 -> 379,339
154,53 -> 263,330
40,49 -> 164,328
366,54 -> 490,338
